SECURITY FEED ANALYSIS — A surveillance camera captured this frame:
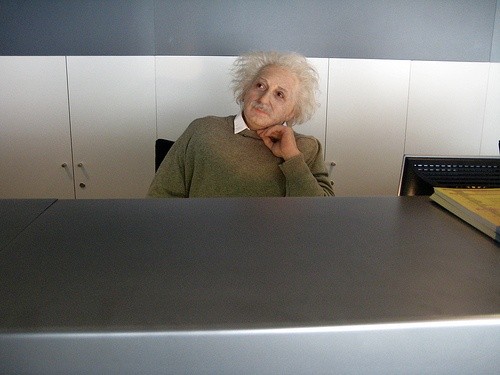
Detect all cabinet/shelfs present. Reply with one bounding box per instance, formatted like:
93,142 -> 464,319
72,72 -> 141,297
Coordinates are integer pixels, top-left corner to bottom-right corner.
0,56 -> 500,200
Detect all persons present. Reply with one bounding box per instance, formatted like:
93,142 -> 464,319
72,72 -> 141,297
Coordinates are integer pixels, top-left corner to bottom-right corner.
145,49 -> 336,198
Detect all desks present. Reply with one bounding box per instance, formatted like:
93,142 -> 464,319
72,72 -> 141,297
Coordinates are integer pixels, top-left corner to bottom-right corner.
0,199 -> 500,375
0,198 -> 58,250
